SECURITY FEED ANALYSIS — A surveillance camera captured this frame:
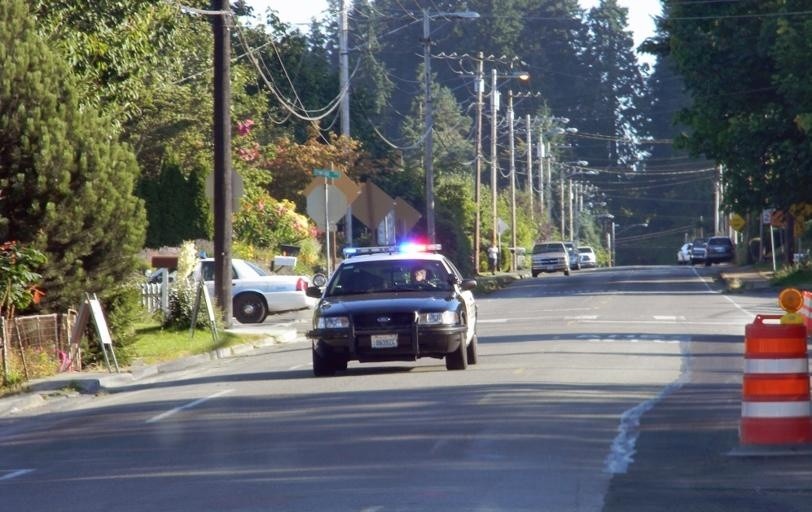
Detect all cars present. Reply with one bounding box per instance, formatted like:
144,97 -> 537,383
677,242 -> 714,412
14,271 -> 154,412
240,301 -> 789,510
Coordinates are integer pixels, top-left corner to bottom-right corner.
678,236 -> 734,265
146,258 -> 313,324
312,242 -> 480,375
531,242 -> 597,277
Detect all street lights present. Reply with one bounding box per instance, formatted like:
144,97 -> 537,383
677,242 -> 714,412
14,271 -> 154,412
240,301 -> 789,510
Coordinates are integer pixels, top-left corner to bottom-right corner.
474,51 -> 529,122
524,112 -> 649,238
337,3 -> 480,79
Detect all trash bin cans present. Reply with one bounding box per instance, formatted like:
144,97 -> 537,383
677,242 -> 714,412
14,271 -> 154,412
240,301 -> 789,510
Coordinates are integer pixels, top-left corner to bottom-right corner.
508,248 -> 525,270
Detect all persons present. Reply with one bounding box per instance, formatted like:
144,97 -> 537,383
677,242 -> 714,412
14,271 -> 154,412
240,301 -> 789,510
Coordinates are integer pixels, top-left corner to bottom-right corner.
487,241 -> 498,275
412,267 -> 437,287
